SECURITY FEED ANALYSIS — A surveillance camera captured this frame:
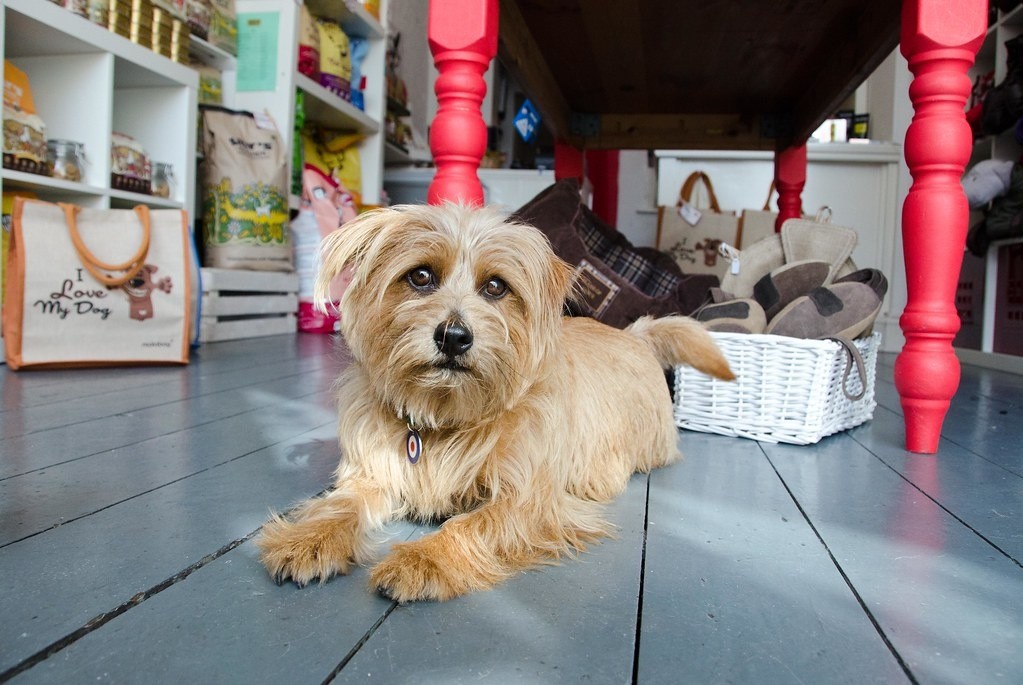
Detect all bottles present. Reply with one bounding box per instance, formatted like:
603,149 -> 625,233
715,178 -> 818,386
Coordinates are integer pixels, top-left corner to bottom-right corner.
47,139 -> 86,181
150,163 -> 176,197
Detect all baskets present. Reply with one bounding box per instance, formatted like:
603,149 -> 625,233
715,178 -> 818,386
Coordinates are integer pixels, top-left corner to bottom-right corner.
673,325 -> 881,445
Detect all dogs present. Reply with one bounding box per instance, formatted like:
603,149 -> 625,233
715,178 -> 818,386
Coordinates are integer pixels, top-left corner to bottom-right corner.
250,198 -> 740,605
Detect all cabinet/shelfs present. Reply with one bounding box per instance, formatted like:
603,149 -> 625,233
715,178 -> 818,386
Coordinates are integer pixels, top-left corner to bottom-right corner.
951,0 -> 1023,374
0,0 -> 516,341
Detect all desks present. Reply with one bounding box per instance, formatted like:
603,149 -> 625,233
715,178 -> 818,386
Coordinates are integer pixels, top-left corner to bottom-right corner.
425,0 -> 988,457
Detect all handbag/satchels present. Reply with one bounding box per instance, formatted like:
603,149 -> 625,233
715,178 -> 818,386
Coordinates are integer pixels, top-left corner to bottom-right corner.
651,171 -> 817,280
4,195 -> 194,365
504,181 -> 722,331
